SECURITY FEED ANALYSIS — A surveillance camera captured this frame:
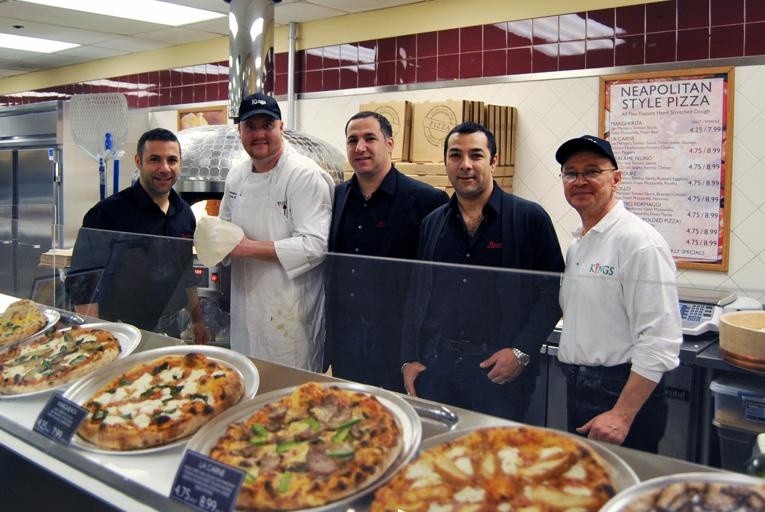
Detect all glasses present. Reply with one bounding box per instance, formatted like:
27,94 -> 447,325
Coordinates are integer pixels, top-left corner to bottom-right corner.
560,168 -> 618,180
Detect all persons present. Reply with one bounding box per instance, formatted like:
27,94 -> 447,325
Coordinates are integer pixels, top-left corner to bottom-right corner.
558,134 -> 683,454
397,121 -> 566,424
325,111 -> 451,391
64,129 -> 210,346
217,93 -> 336,374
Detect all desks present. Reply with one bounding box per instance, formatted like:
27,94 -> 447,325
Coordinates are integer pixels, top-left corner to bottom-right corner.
694,340 -> 763,477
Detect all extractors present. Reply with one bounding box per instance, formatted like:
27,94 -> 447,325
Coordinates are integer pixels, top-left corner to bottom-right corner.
169,120 -> 346,197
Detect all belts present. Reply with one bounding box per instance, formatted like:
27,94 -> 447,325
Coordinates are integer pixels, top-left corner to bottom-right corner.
421,340 -> 495,360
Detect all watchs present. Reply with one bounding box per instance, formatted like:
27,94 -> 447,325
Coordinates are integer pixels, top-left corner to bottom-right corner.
511,347 -> 530,366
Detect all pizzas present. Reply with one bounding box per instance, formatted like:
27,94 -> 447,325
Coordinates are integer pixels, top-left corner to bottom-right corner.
208,385 -> 403,512
620,479 -> 765,511
73,353 -> 245,450
0,329 -> 122,394
0,299 -> 46,345
371,427 -> 616,511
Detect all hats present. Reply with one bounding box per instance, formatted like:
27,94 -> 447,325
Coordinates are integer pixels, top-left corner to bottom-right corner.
239,93 -> 282,120
555,135 -> 619,168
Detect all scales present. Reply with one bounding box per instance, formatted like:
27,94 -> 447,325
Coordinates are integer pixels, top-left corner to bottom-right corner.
677,288 -> 763,342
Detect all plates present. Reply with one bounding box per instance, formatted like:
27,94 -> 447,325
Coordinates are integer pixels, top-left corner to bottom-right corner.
370,423 -> 641,512
0,320 -> 142,400
184,380 -> 424,512
0,308 -> 63,350
597,468 -> 765,512
60,344 -> 261,457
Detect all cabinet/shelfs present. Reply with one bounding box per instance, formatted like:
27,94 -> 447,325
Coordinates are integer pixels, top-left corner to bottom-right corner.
507,294 -> 721,461
0,219 -> 765,512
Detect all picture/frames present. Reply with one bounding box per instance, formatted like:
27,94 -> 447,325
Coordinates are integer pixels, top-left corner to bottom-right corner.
598,64 -> 736,274
